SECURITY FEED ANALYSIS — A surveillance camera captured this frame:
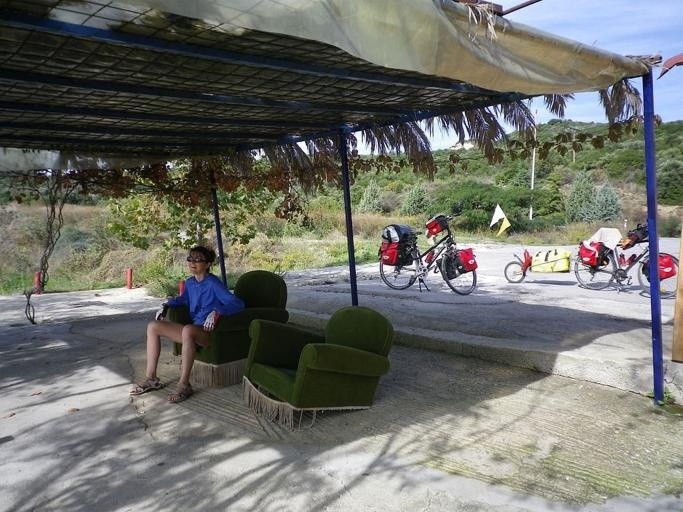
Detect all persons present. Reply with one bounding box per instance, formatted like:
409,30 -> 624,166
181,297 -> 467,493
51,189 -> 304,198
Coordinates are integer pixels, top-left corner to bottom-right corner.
129,247 -> 246,404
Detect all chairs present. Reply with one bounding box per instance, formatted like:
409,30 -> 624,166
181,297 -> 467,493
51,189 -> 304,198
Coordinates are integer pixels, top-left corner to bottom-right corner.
169,270 -> 289,387
244,306 -> 395,431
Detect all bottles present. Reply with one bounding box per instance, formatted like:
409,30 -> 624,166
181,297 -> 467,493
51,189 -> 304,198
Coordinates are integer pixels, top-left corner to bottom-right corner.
424,250 -> 434,264
617,252 -> 636,266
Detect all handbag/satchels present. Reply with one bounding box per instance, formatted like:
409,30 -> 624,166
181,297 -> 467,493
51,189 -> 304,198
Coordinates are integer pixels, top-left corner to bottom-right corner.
436,248 -> 478,281
577,240 -> 604,267
379,224 -> 416,266
426,213 -> 447,236
641,253 -> 678,284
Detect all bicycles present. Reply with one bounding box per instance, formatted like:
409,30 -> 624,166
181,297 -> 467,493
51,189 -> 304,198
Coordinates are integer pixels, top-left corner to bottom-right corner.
377,211 -> 479,295
576,220 -> 679,299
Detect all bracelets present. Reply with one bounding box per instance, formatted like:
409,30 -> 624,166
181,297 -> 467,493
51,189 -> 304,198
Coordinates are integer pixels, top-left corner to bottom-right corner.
161,303 -> 170,308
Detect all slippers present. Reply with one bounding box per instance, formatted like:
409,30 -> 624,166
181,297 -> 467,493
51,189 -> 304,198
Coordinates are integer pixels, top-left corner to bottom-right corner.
128,376 -> 163,396
166,382 -> 193,402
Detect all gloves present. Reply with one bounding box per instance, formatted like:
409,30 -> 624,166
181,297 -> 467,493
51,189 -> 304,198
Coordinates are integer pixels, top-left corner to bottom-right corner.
202,309 -> 221,333
153,302 -> 170,321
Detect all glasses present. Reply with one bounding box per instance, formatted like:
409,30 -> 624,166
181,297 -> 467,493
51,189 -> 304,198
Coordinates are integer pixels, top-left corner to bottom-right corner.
186,256 -> 207,263
